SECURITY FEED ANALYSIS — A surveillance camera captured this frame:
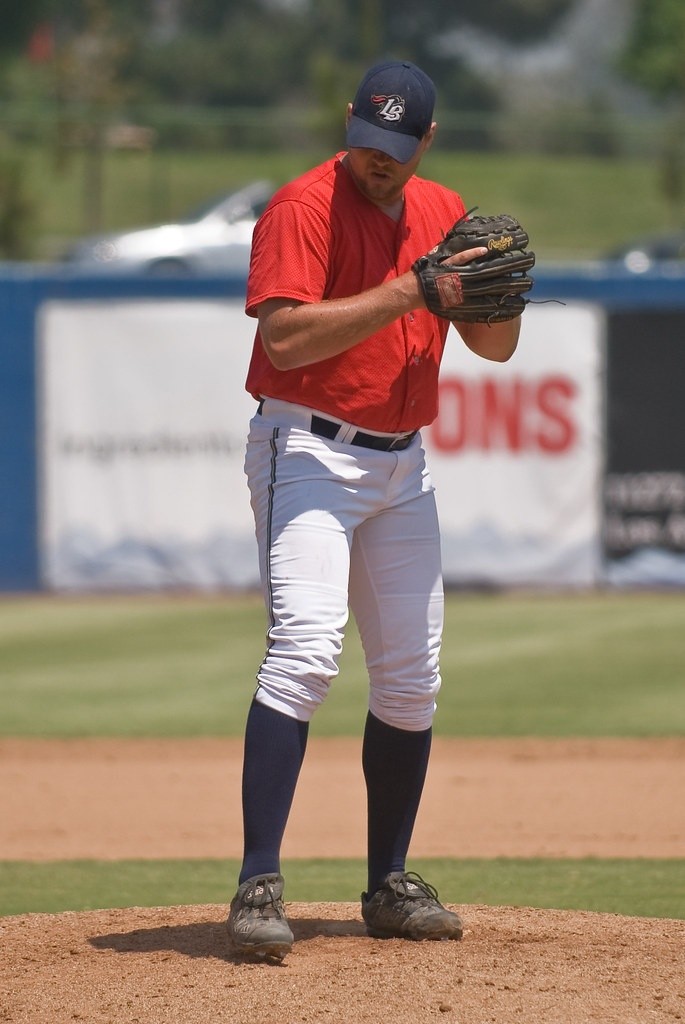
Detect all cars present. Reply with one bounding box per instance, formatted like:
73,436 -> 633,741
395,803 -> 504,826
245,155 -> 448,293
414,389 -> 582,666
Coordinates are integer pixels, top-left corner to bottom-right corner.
89,179 -> 275,277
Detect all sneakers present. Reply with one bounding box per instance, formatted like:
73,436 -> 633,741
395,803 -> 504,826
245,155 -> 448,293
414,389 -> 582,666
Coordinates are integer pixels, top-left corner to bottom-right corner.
361,871 -> 465,939
226,872 -> 294,956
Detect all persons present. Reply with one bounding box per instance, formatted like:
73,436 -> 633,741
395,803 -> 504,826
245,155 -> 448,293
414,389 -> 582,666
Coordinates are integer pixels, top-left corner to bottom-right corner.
228,61 -> 535,958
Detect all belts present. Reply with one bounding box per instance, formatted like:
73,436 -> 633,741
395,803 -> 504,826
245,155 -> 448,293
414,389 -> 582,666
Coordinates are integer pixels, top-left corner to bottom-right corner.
258,401 -> 418,452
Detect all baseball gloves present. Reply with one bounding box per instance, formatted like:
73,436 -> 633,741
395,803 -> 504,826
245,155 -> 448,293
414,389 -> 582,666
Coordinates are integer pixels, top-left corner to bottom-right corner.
410,205 -> 569,330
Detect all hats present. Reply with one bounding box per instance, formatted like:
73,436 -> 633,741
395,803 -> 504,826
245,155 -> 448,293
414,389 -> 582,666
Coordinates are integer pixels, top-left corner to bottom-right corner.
346,60 -> 435,163
